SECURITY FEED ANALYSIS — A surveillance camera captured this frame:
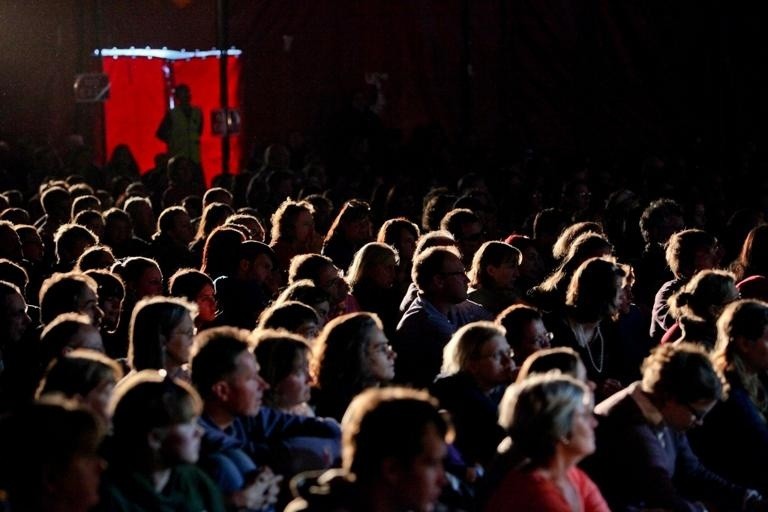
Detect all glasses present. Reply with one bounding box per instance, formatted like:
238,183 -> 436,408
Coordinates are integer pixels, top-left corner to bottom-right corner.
174,328 -> 198,338
534,332 -> 554,346
371,346 -> 392,354
494,349 -> 514,362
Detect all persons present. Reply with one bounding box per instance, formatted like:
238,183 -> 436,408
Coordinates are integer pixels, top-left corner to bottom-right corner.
156,84 -> 204,190
1,130 -> 768,512
326,87 -> 372,170
